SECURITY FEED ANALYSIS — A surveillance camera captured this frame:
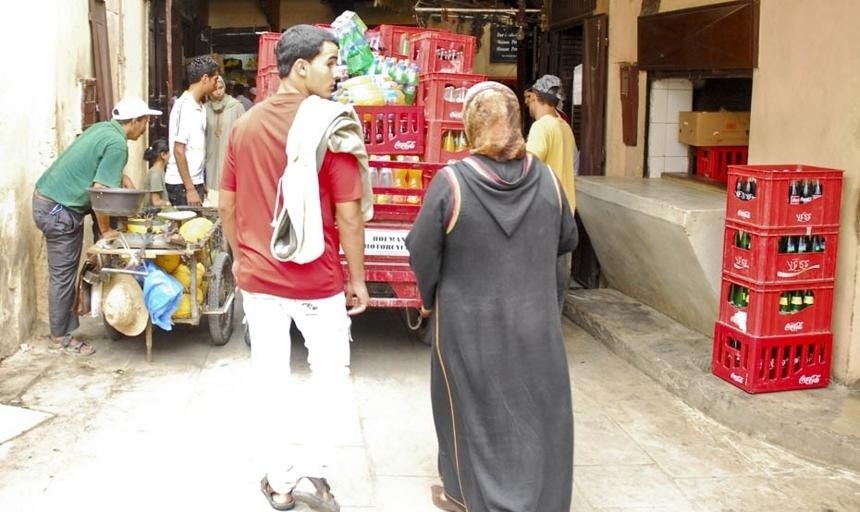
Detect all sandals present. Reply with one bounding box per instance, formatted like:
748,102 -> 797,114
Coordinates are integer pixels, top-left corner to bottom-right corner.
293,477 -> 339,512
259,476 -> 293,512
50,334 -> 97,359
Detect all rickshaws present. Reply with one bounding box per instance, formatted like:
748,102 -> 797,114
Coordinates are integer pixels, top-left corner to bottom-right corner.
82,203 -> 235,345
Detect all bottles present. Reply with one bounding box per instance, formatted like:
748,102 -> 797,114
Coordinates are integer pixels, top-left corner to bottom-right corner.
735,235 -> 751,247
778,293 -> 789,314
798,234 -> 808,252
449,84 -> 474,98
732,287 -> 748,304
750,177 -> 757,197
458,127 -> 467,150
788,179 -> 800,204
408,168 -> 424,202
815,236 -> 825,255
786,231 -> 795,256
816,177 -> 831,201
338,75 -> 405,104
724,337 -> 742,360
397,164 -> 407,203
805,291 -> 815,304
368,162 -> 378,205
356,111 -> 420,151
434,38 -> 463,73
381,167 -> 392,203
445,130 -> 454,149
760,347 -> 824,376
803,177 -> 811,200
734,179 -> 744,200
794,291 -> 802,310
340,20 -> 375,71
383,51 -> 419,102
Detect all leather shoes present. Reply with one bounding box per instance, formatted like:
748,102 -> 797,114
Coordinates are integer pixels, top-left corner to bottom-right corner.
431,484 -> 462,512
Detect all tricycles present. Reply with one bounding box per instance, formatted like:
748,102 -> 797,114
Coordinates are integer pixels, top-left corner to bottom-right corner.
245,223 -> 432,347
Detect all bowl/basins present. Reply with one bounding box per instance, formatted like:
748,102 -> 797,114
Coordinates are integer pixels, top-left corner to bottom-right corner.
86,186 -> 147,217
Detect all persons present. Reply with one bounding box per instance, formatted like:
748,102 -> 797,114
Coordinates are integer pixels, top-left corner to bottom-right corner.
407,79 -> 580,511
32,99 -> 164,353
202,74 -> 246,206
233,83 -> 253,111
218,26 -> 370,512
161,54 -> 218,206
523,72 -> 579,221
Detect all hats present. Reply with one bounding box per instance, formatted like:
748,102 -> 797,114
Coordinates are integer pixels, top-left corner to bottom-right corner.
112,97 -> 162,119
101,273 -> 150,337
532,75 -> 564,97
